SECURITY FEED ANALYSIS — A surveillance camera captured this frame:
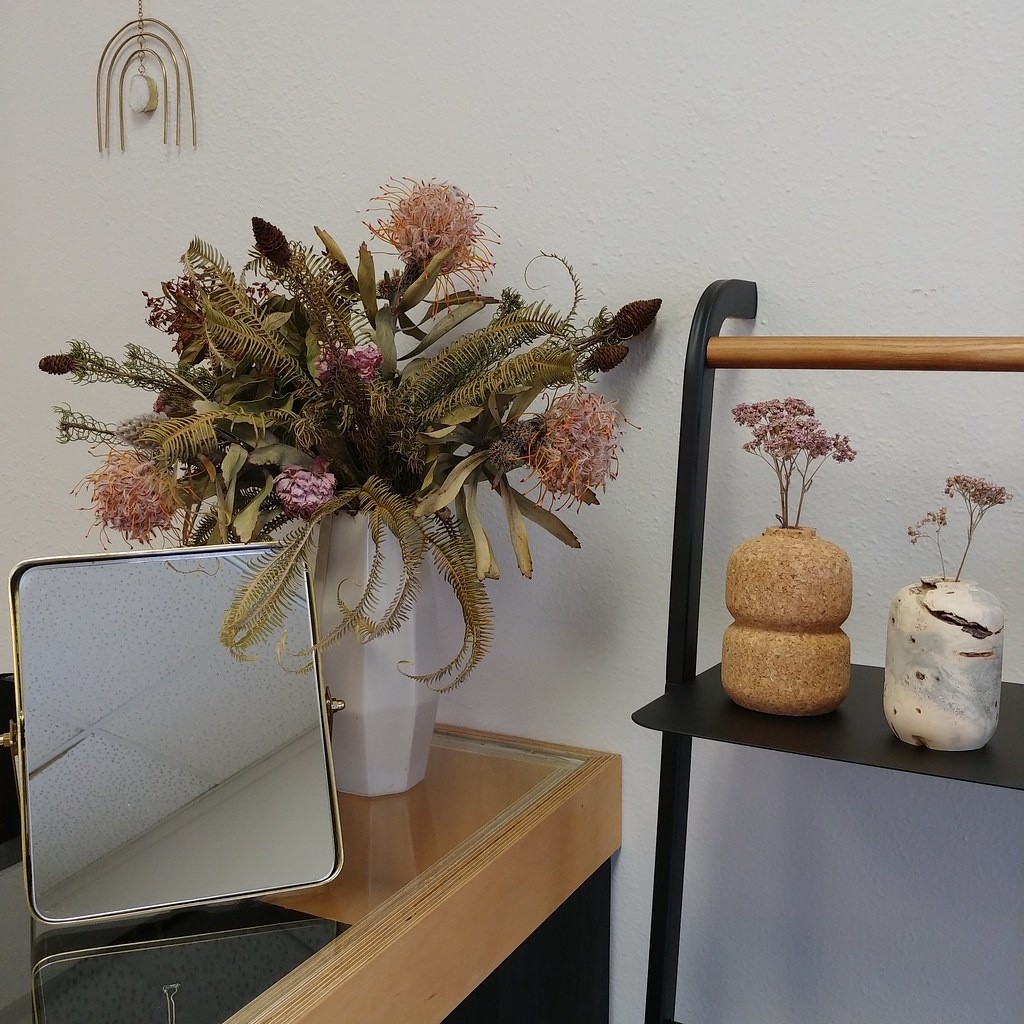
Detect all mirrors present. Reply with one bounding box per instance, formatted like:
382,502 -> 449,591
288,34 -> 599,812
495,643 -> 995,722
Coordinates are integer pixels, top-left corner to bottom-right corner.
0,541 -> 345,928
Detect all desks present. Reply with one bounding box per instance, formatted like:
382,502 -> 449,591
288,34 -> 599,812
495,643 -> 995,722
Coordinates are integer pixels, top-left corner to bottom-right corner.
0,722 -> 623,1024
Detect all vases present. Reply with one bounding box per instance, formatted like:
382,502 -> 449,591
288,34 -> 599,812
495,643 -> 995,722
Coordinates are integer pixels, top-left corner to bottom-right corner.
883,576 -> 1005,751
722,527 -> 852,717
314,512 -> 438,797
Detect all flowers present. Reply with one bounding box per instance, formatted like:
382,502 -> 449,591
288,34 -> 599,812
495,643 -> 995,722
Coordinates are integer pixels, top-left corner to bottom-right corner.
730,397 -> 857,527
907,474 -> 1016,581
36,176 -> 661,695
